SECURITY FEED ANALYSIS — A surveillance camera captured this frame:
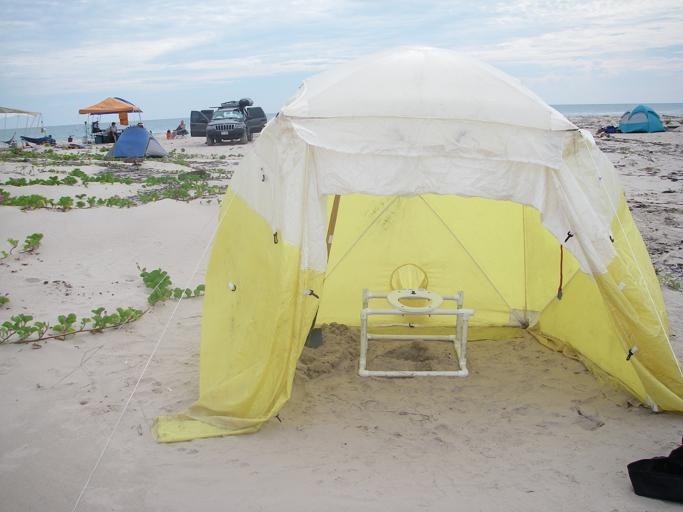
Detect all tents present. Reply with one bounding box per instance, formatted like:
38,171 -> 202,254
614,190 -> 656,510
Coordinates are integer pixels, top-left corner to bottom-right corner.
105,126 -> 170,158
618,103 -> 667,132
156,42 -> 683,444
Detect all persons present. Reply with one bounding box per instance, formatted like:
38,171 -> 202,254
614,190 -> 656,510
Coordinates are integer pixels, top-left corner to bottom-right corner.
110,121 -> 117,143
165,129 -> 173,139
176,119 -> 185,136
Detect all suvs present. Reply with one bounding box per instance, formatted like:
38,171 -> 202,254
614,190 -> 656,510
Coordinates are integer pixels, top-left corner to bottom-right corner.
190,102 -> 267,146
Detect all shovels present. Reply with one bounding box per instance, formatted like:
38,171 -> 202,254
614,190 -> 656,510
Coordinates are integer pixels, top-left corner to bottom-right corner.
302,195 -> 342,348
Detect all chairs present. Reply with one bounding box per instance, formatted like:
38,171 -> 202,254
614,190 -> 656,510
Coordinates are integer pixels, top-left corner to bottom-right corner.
173,124 -> 188,138
